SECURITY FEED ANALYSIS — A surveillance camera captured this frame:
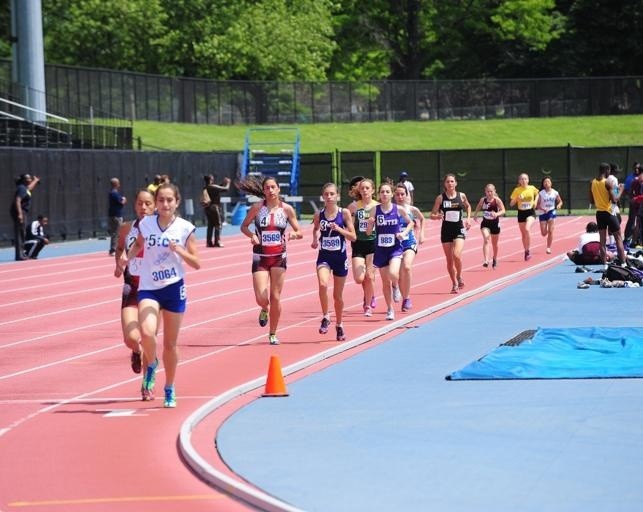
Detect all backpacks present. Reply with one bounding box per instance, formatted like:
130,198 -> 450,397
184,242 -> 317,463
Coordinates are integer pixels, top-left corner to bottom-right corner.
200,187 -> 212,209
607,265 -> 643,283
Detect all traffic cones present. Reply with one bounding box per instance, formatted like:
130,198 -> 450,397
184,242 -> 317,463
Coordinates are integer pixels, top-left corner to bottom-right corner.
262,354 -> 289,396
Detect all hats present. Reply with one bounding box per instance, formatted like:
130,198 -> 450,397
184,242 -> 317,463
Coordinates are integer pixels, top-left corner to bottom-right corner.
400,172 -> 407,177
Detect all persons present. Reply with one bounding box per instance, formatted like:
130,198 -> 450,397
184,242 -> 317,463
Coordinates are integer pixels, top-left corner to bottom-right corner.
509,174 -> 539,261
147,175 -> 163,193
346,178 -> 382,317
204,174 -> 231,247
114,188 -> 157,374
630,165 -> 643,196
23,216 -> 49,258
366,182 -> 415,321
605,163 -> 621,248
128,184 -> 201,408
393,183 -> 426,313
621,164 -> 643,237
311,182 -> 357,341
566,221 -> 618,265
430,174 -> 472,294
537,176 -> 563,254
10,174 -> 40,260
161,174 -> 171,186
474,184 -> 505,270
107,178 -> 127,255
589,162 -> 627,269
399,173 -> 415,206
240,177 -> 303,346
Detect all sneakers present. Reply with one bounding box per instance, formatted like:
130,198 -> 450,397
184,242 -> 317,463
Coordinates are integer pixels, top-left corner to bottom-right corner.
576,267 -> 585,272
258,310 -> 268,328
362,306 -> 371,318
268,334 -> 278,345
130,350 -> 142,373
143,360 -> 158,390
482,262 -> 488,268
577,284 -> 587,289
525,252 -> 530,261
370,297 -> 376,309
214,243 -> 222,246
583,266 -> 591,272
401,298 -> 411,313
206,242 -> 213,248
318,319 -> 330,333
335,326 -> 345,340
163,384 -> 175,407
393,287 -> 400,302
142,385 -> 153,401
584,279 -> 598,285
450,285 -> 459,295
458,279 -> 465,289
546,248 -> 550,253
492,260 -> 497,268
385,309 -> 394,321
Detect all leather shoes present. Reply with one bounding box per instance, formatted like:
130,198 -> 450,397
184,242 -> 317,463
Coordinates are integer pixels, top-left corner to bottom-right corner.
15,256 -> 26,260
